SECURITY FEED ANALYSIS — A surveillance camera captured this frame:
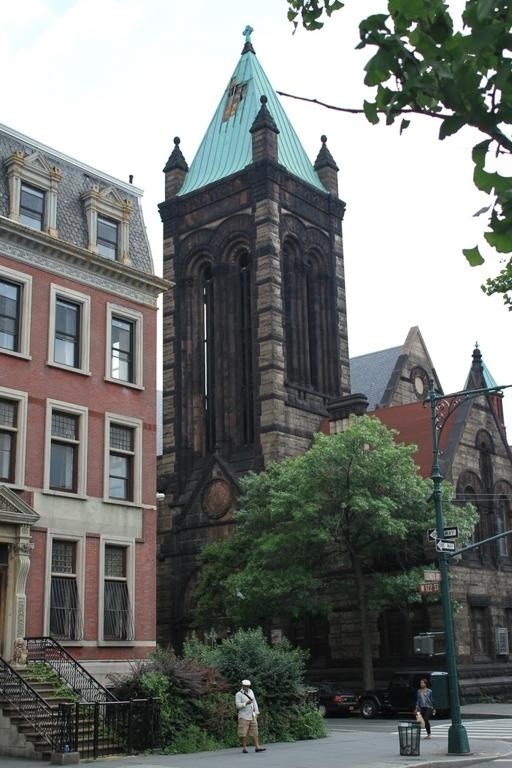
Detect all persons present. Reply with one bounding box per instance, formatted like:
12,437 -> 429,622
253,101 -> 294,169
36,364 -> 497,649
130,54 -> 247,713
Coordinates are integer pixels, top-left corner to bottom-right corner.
234,680 -> 266,753
414,679 -> 436,738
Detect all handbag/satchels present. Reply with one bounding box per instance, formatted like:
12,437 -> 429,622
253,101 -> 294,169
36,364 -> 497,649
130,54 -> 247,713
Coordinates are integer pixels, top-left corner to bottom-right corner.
416,711 -> 425,729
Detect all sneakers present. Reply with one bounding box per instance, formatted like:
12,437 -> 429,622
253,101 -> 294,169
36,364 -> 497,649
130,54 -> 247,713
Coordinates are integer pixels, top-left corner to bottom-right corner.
424,734 -> 432,739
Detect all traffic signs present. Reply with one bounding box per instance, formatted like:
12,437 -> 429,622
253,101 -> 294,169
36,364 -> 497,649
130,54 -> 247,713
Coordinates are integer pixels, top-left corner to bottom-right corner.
435,540 -> 455,553
428,527 -> 457,541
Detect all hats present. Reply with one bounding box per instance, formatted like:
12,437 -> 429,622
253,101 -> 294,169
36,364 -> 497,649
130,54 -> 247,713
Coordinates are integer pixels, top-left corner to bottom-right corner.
242,679 -> 252,685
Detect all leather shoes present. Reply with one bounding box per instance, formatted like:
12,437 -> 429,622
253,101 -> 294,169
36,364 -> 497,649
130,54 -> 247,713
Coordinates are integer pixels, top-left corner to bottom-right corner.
242,749 -> 248,753
255,747 -> 266,752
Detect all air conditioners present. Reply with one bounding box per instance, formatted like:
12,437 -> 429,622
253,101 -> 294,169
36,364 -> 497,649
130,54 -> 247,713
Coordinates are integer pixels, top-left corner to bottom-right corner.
413,636 -> 435,655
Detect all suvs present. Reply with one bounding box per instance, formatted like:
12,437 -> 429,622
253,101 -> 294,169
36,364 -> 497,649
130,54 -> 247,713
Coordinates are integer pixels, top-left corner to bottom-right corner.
360,670 -> 450,716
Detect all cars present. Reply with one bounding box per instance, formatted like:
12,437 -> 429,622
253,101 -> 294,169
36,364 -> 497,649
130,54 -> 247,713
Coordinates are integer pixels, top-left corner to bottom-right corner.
310,682 -> 360,717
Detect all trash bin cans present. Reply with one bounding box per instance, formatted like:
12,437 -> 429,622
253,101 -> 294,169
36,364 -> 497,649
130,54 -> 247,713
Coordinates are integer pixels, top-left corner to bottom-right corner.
398,720 -> 421,756
